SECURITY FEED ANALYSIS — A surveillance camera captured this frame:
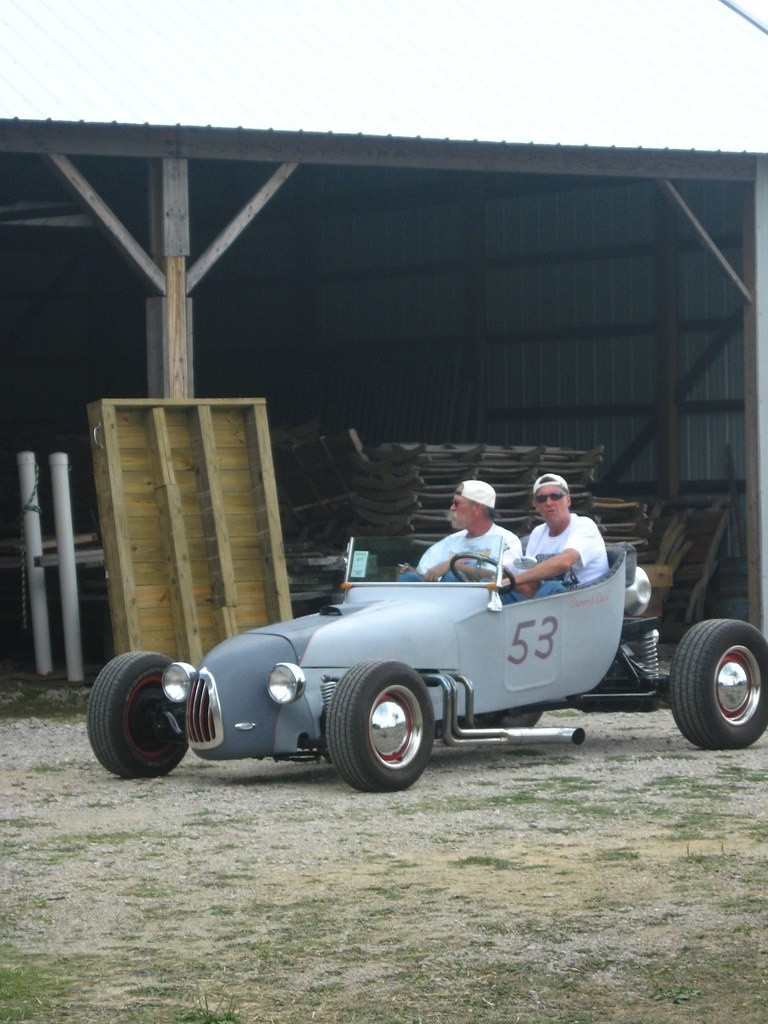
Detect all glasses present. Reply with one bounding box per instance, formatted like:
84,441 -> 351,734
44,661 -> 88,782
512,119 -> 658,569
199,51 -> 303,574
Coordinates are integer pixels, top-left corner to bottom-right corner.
535,492 -> 566,503
453,501 -> 472,507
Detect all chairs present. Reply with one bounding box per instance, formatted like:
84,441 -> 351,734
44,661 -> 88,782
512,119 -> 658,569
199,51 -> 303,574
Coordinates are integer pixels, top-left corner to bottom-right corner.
276,429 -> 734,641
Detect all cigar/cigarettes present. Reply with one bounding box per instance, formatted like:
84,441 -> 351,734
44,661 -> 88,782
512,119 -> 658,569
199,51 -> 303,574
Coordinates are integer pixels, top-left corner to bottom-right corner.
398,563 -> 409,569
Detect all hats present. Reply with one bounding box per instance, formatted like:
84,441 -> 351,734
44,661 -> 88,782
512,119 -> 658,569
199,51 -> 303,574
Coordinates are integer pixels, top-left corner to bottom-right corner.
533,473 -> 569,496
456,480 -> 503,520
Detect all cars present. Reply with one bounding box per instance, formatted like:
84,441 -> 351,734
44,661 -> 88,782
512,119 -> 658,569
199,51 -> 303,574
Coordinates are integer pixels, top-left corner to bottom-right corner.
88,536 -> 767,794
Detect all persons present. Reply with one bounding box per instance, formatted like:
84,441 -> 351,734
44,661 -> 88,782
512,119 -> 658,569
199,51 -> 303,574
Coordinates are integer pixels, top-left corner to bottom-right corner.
500,471 -> 610,605
396,477 -> 524,583
